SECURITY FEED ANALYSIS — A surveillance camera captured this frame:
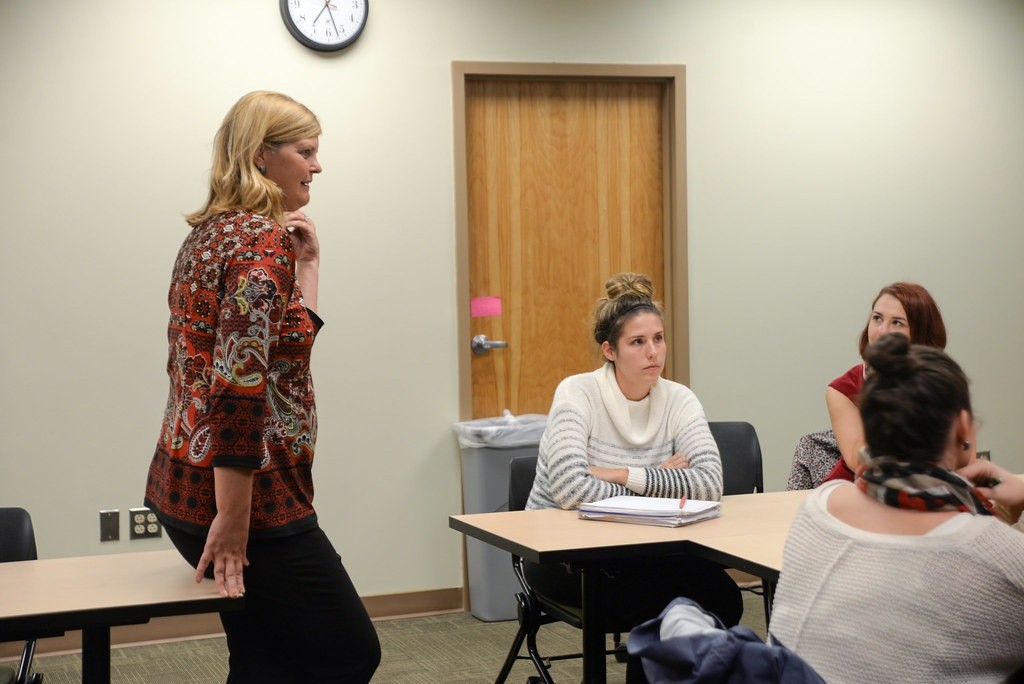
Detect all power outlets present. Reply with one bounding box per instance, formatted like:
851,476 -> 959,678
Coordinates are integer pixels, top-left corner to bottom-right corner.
129,509 -> 162,539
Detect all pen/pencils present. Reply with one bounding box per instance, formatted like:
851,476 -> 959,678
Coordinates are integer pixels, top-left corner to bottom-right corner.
679,496 -> 687,509
975,476 -> 1003,488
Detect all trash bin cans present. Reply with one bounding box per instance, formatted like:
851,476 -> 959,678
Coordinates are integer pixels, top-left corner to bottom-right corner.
453,412 -> 550,623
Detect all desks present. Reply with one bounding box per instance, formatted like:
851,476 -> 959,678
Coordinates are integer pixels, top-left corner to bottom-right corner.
449,488 -> 815,684
0,550 -> 227,684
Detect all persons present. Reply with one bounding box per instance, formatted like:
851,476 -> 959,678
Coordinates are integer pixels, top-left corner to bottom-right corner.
519,274 -> 744,630
766,332 -> 1024,684
818,282 -> 947,486
144,89 -> 382,684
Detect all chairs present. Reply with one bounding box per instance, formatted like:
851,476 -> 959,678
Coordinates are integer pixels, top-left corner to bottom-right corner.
494,455 -> 744,684
0,508 -> 44,684
708,421 -> 780,632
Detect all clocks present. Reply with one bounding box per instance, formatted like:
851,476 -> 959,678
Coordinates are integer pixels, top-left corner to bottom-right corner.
280,0 -> 370,52
